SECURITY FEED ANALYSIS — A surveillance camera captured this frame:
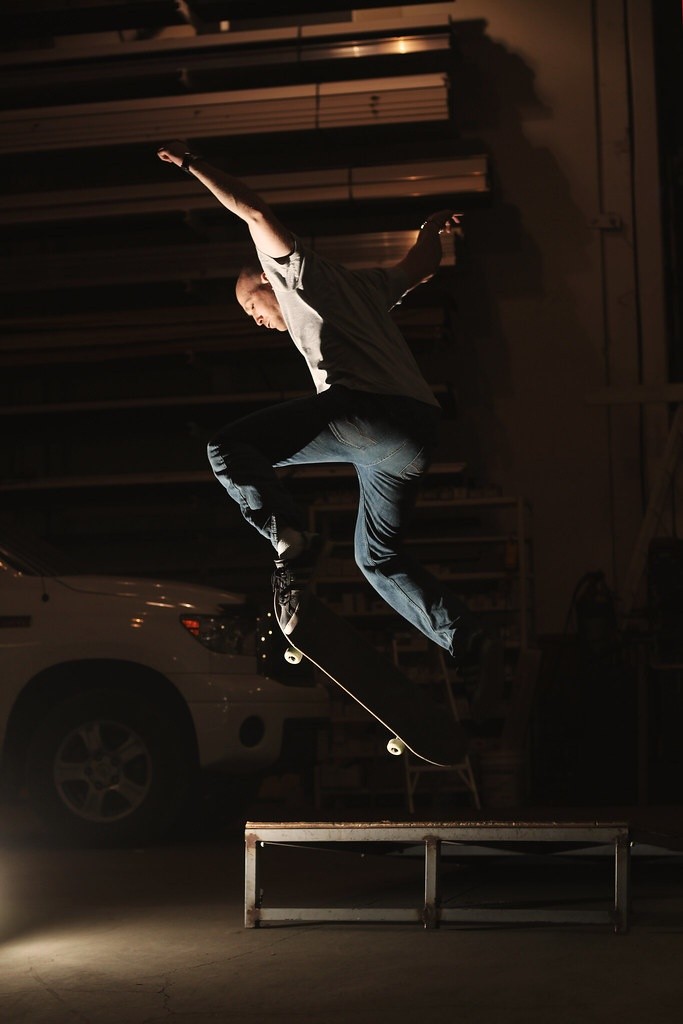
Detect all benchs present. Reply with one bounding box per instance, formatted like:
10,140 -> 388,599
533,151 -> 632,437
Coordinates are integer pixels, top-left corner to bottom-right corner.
242,819 -> 632,933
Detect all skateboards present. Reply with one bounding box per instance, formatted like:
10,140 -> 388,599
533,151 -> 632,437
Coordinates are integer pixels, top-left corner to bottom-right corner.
272,587 -> 469,767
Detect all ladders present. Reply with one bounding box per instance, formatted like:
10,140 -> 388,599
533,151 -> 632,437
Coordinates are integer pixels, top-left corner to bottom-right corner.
393,637 -> 480,814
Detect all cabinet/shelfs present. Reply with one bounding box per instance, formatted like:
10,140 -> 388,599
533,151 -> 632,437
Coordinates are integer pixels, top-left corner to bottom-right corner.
308,497 -> 533,813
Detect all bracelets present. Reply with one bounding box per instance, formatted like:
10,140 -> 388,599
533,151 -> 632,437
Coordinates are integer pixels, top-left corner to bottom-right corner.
181,151 -> 200,174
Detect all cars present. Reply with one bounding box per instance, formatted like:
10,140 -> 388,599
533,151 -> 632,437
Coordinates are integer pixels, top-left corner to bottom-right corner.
0,525 -> 332,852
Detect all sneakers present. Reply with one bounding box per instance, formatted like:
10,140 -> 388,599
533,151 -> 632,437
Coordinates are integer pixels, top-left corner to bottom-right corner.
270,528 -> 328,637
452,619 -> 506,730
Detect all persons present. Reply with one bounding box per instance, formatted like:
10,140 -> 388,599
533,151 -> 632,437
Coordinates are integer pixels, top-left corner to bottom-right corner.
156,138 -> 505,720
